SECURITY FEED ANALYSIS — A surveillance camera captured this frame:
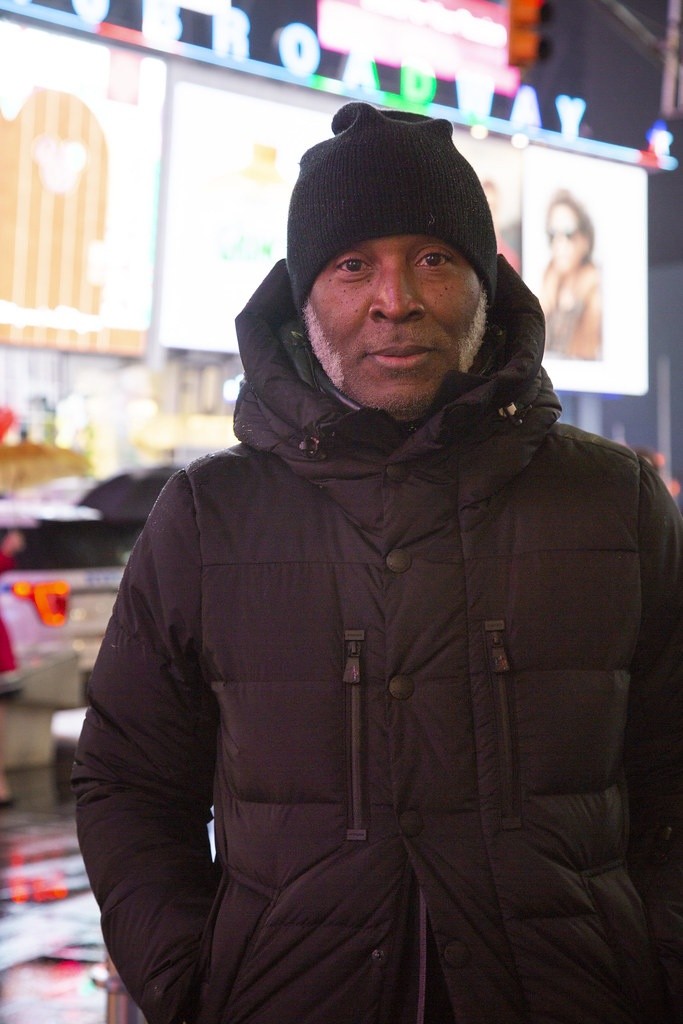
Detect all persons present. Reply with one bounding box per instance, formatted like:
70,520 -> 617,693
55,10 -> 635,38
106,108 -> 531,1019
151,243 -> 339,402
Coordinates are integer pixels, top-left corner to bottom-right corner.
62,102 -> 683,1023
523,185 -> 605,363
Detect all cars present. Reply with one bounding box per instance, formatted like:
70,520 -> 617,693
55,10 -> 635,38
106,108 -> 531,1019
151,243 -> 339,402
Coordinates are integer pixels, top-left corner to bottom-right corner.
0,496 -> 126,691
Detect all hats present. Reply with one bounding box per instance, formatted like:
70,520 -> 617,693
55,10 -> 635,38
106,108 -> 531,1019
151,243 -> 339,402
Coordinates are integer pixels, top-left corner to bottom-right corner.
286,103 -> 496,306
